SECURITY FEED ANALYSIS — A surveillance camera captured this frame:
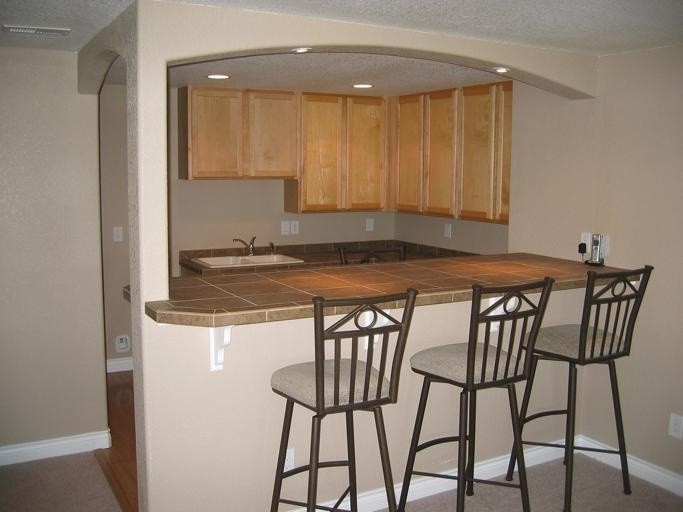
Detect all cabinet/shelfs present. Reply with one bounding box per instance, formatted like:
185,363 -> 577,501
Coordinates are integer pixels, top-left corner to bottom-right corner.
284,90 -> 385,215
458,80 -> 511,225
175,83 -> 298,181
393,85 -> 457,218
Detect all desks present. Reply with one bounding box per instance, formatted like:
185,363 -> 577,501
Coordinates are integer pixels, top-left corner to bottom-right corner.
123,244 -> 638,512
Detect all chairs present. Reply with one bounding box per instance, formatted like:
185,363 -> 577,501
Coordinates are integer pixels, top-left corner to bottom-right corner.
266,286 -> 419,511
332,242 -> 406,265
503,263 -> 657,512
398,270 -> 558,512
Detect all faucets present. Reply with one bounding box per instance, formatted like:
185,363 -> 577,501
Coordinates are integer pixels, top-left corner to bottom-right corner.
247,234 -> 258,256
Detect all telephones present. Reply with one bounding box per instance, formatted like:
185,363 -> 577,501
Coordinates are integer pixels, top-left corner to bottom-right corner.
583,234 -> 604,267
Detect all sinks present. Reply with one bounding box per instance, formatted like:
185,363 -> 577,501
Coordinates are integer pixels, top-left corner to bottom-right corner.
190,253 -> 305,269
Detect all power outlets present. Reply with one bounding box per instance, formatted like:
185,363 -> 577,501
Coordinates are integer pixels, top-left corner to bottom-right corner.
579,230 -> 591,255
